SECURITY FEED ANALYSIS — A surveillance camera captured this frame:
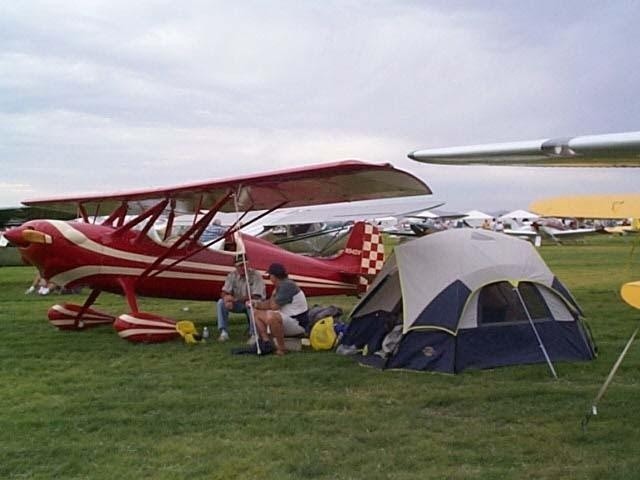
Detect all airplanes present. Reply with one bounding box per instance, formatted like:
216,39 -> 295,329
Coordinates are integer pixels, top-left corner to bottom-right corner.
0,159 -> 433,345
407,130 -> 640,169
502,220 -> 605,246
403,213 -> 472,237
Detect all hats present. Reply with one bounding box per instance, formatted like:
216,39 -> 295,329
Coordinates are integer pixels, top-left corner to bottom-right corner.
234,253 -> 249,266
266,262 -> 286,275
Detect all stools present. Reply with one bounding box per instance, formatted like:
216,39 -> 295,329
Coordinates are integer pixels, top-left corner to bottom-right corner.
272,334 -> 303,351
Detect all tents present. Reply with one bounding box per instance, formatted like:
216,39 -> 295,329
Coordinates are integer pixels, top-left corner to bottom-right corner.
332,228 -> 600,377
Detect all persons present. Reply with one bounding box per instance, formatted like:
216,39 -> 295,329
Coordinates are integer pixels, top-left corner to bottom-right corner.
481,218 -> 504,232
217,253 -> 308,354
199,219 -> 232,242
25,272 -> 57,295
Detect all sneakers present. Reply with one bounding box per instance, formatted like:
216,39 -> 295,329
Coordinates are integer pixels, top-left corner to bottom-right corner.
217,328 -> 290,356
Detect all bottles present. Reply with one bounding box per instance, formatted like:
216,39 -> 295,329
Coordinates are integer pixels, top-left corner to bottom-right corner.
202,326 -> 209,338
